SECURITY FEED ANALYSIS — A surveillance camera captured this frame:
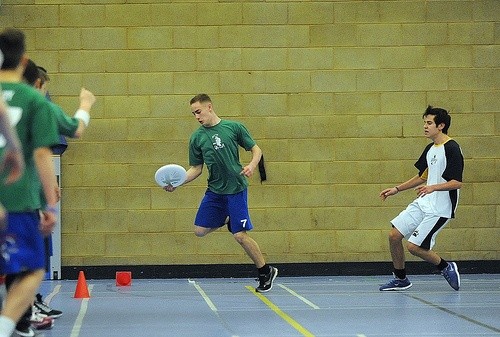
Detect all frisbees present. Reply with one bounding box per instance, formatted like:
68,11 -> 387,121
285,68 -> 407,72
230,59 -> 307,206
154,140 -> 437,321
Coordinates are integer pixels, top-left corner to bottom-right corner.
154,164 -> 188,190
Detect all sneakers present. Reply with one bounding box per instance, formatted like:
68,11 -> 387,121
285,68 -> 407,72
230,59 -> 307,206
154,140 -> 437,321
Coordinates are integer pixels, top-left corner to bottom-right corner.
30,305 -> 54,329
255,265 -> 279,293
379,271 -> 413,291
33,293 -> 63,319
440,261 -> 460,291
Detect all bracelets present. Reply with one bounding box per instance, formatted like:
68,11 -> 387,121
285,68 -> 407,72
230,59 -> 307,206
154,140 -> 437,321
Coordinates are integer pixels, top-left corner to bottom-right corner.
395,186 -> 400,192
44,205 -> 57,214
74,109 -> 90,126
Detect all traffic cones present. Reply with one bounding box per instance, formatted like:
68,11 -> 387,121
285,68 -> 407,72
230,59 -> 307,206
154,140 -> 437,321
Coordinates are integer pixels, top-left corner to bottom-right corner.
73,270 -> 91,298
116,271 -> 131,286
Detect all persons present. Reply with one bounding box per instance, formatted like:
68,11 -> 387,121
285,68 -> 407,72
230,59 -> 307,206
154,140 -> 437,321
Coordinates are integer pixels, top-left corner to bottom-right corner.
28,67 -> 55,331
23,57 -> 54,329
0,49 -> 24,227
32,65 -> 62,319
163,93 -> 278,292
0,29 -> 58,337
379,105 -> 464,291
